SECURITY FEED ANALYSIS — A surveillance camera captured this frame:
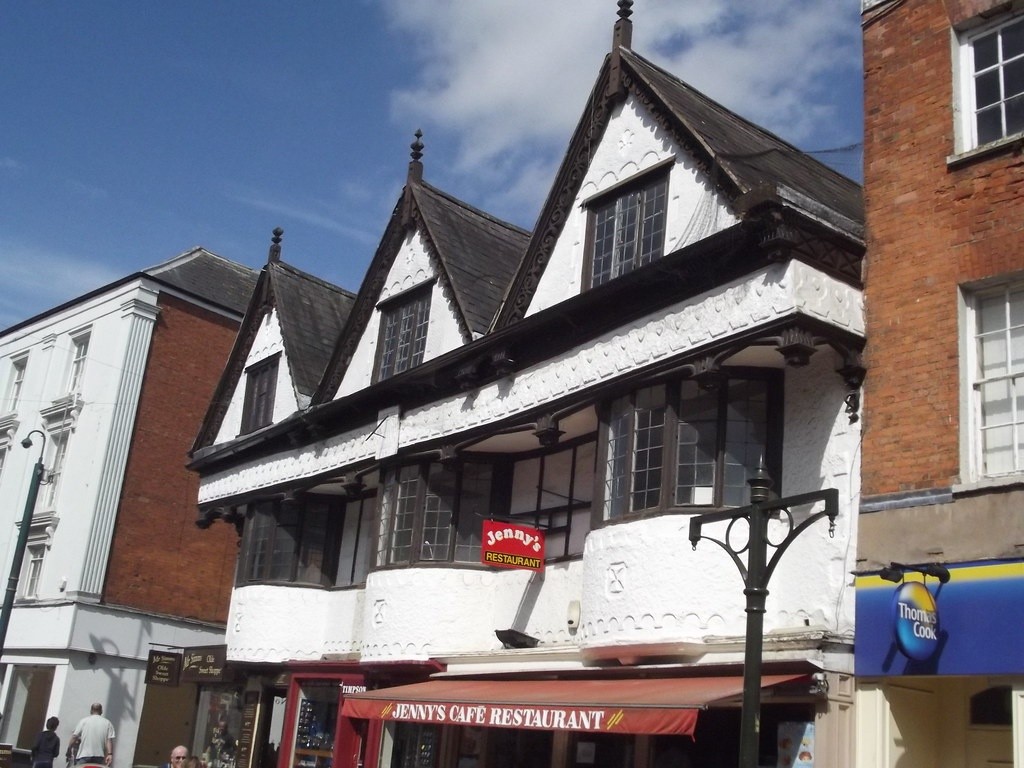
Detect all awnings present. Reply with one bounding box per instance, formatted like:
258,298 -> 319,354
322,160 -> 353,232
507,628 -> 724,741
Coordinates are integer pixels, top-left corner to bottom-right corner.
341,674 -> 813,741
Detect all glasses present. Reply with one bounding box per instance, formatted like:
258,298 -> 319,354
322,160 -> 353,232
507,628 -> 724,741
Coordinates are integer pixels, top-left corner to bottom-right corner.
173,755 -> 185,760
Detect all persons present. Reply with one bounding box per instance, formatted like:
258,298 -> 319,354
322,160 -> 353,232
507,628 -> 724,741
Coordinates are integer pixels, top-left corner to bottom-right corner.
204,720 -> 237,761
30,716 -> 61,768
160,745 -> 204,767
66,703 -> 116,767
